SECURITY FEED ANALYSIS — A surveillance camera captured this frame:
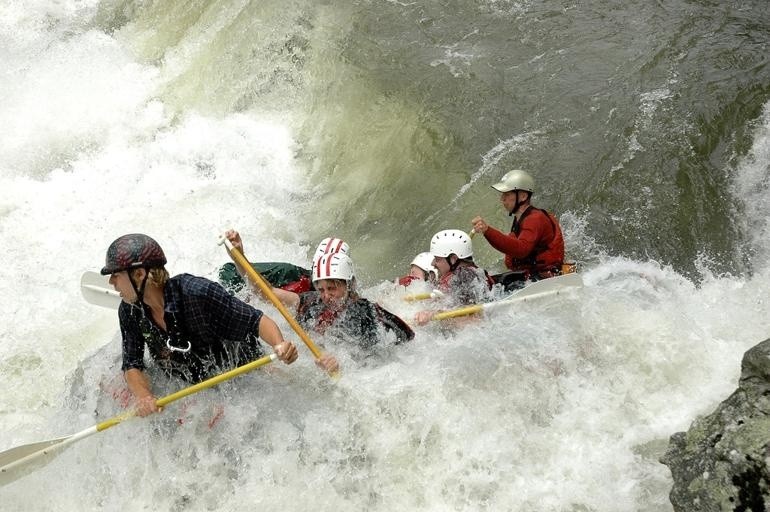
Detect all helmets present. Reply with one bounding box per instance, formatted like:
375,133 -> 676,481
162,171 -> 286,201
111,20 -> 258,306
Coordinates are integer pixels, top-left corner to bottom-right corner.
313,238 -> 350,262
491,169 -> 535,195
430,229 -> 473,259
101,233 -> 168,276
311,251 -> 356,290
409,251 -> 439,281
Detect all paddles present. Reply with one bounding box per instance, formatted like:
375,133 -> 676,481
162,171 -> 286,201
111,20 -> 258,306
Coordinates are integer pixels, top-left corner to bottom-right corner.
80,271 -> 124,309
428,273 -> 584,321
0,344 -> 282,487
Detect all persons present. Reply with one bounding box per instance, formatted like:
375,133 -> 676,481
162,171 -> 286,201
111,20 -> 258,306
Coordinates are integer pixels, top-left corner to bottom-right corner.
389,251 -> 440,289
411,227 -> 501,342
217,236 -> 417,346
216,227 -> 402,382
99,231 -> 300,421
469,168 -> 565,294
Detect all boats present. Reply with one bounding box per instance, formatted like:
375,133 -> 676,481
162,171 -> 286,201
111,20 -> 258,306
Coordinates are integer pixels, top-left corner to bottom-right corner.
54,337 -> 370,492
